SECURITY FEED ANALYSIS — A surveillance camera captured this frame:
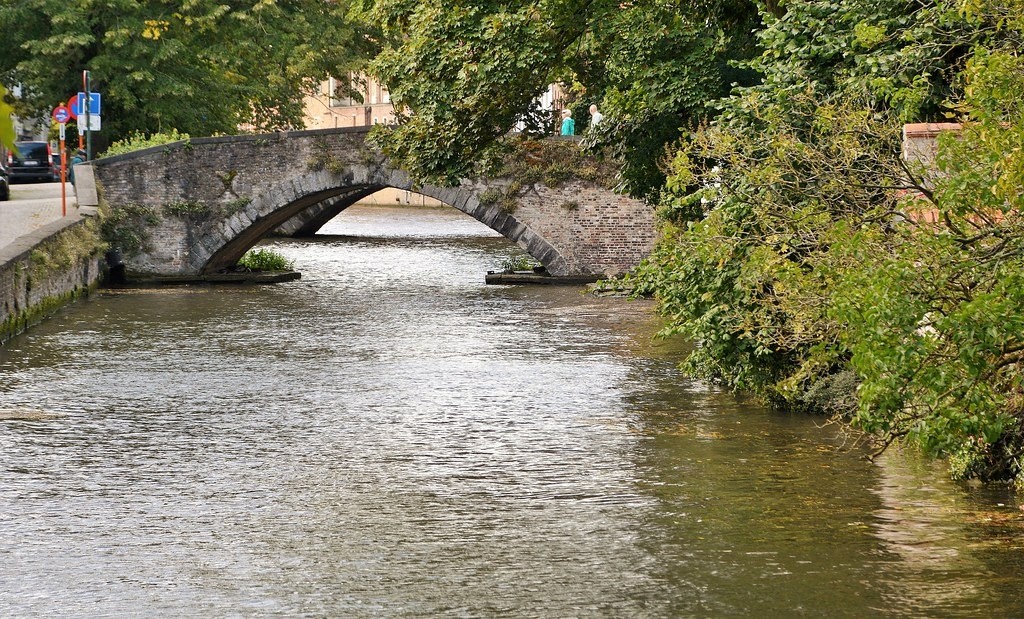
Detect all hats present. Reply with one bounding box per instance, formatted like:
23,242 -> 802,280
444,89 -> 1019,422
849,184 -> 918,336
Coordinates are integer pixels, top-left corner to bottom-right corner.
77,150 -> 86,155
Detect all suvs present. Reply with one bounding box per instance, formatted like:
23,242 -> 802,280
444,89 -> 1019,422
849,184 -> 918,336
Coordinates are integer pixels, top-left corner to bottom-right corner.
6,141 -> 55,183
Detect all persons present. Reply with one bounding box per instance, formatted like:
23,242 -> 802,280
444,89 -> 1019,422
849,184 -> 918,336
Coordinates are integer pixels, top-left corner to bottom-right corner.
589,105 -> 604,129
71,149 -> 88,210
561,109 -> 575,136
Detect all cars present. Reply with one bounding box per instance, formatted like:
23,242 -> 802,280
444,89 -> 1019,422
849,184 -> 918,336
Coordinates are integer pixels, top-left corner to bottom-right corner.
51,153 -> 68,181
0,161 -> 12,200
